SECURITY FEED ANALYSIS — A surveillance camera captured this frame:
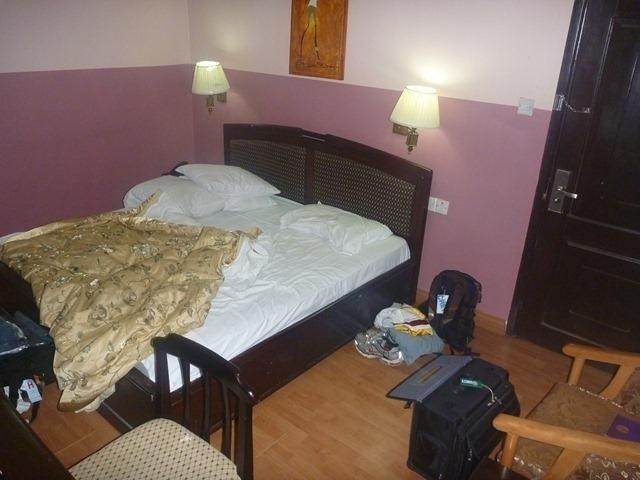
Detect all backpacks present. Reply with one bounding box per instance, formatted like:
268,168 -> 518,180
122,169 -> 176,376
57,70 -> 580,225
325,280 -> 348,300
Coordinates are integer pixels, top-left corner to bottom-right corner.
418,269 -> 482,355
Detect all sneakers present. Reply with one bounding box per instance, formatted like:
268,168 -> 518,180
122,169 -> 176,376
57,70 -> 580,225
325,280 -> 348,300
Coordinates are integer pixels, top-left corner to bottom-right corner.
354,331 -> 403,367
366,327 -> 383,337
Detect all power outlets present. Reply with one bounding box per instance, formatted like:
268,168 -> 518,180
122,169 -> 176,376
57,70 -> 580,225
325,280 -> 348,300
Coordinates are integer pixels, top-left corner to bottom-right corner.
434,198 -> 449,216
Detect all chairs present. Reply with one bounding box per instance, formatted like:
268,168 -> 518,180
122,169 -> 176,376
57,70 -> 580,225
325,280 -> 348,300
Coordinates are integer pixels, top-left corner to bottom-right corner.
67,333 -> 258,480
488,342 -> 640,480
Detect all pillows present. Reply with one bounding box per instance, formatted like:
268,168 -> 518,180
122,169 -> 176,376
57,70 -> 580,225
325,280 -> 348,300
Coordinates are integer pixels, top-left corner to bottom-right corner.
281,202 -> 394,255
174,154 -> 282,200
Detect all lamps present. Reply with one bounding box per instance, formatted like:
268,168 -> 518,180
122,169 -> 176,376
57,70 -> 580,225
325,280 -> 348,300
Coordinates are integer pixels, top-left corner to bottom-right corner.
388,85 -> 440,151
191,61 -> 230,114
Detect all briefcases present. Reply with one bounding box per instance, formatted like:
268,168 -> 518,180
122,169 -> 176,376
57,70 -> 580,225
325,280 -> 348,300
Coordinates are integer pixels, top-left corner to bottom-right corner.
386,355 -> 521,480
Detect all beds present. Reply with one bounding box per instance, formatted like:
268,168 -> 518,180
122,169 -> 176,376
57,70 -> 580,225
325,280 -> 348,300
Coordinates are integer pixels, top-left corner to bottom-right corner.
0,123 -> 433,435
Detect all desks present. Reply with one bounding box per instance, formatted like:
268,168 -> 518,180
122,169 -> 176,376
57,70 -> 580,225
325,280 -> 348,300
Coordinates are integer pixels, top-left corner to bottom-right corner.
0,391 -> 76,480
